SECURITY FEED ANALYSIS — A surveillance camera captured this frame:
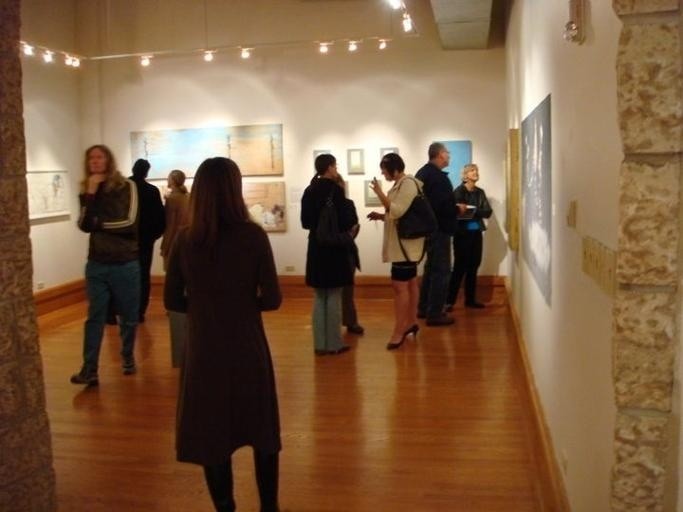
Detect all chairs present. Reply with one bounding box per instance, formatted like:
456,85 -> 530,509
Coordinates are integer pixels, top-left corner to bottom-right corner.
122,355 -> 135,375
347,325 -> 363,334
70,368 -> 98,384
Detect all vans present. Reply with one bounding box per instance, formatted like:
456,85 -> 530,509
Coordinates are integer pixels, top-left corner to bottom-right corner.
387,324 -> 418,349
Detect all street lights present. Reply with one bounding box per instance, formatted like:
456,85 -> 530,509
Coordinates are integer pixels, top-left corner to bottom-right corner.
398,196 -> 437,238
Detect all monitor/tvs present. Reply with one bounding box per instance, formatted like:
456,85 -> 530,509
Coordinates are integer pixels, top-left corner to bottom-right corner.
455,203 -> 477,220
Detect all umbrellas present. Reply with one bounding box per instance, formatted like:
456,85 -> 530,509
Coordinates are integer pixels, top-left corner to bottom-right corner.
416,312 -> 454,326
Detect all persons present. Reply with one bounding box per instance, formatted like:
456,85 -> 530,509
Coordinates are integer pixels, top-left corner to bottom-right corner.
161,157 -> 282,511
157,169 -> 192,276
69,144 -> 142,385
444,164 -> 493,312
333,174 -> 363,335
411,142 -> 468,326
364,153 -> 430,350
300,154 -> 357,355
101,157 -> 167,326
